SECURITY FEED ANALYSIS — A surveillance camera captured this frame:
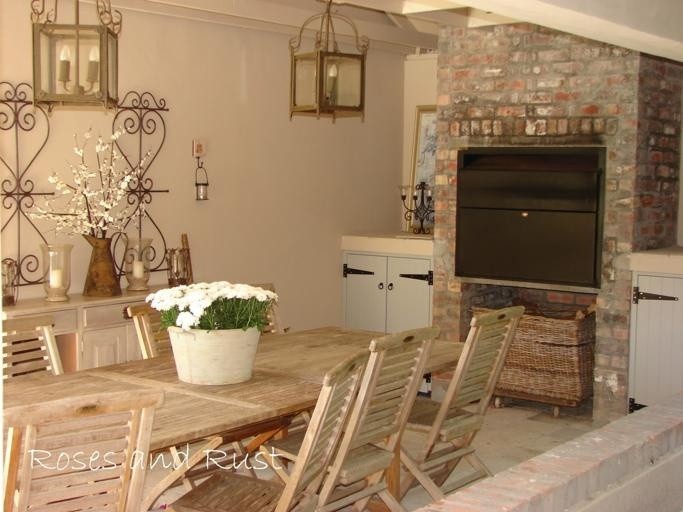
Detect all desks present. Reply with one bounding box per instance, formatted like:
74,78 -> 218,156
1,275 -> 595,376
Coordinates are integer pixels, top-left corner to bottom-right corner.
2,325 -> 464,475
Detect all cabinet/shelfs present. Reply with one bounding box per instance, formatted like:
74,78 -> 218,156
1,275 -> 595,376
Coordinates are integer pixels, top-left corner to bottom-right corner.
2,295 -> 145,381
342,236 -> 434,399
626,272 -> 683,418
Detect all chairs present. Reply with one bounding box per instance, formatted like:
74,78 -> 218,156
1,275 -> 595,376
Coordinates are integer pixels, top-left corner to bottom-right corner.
170,348 -> 369,512
127,304 -> 170,359
259,327 -> 445,512
2,389 -> 164,512
387,306 -> 524,502
245,284 -> 279,334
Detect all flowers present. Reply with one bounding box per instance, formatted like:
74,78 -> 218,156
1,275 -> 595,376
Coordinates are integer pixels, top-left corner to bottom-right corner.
146,281 -> 277,331
24,125 -> 149,236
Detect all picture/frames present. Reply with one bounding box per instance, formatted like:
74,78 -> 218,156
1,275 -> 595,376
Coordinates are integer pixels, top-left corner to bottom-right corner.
405,105 -> 439,240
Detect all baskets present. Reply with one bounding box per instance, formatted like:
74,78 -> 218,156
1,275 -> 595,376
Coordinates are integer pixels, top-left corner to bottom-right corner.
470,302 -> 596,401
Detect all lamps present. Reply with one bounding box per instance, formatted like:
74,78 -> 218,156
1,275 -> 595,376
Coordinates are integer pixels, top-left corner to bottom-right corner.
29,0 -> 121,114
287,0 -> 370,124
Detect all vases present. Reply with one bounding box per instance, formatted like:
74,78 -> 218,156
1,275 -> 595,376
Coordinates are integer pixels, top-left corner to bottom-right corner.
164,248 -> 191,285
167,324 -> 259,386
83,233 -> 121,298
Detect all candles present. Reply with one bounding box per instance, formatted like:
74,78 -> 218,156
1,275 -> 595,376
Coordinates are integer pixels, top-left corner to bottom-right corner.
132,261 -> 145,279
50,269 -> 62,287
402,188 -> 431,197
